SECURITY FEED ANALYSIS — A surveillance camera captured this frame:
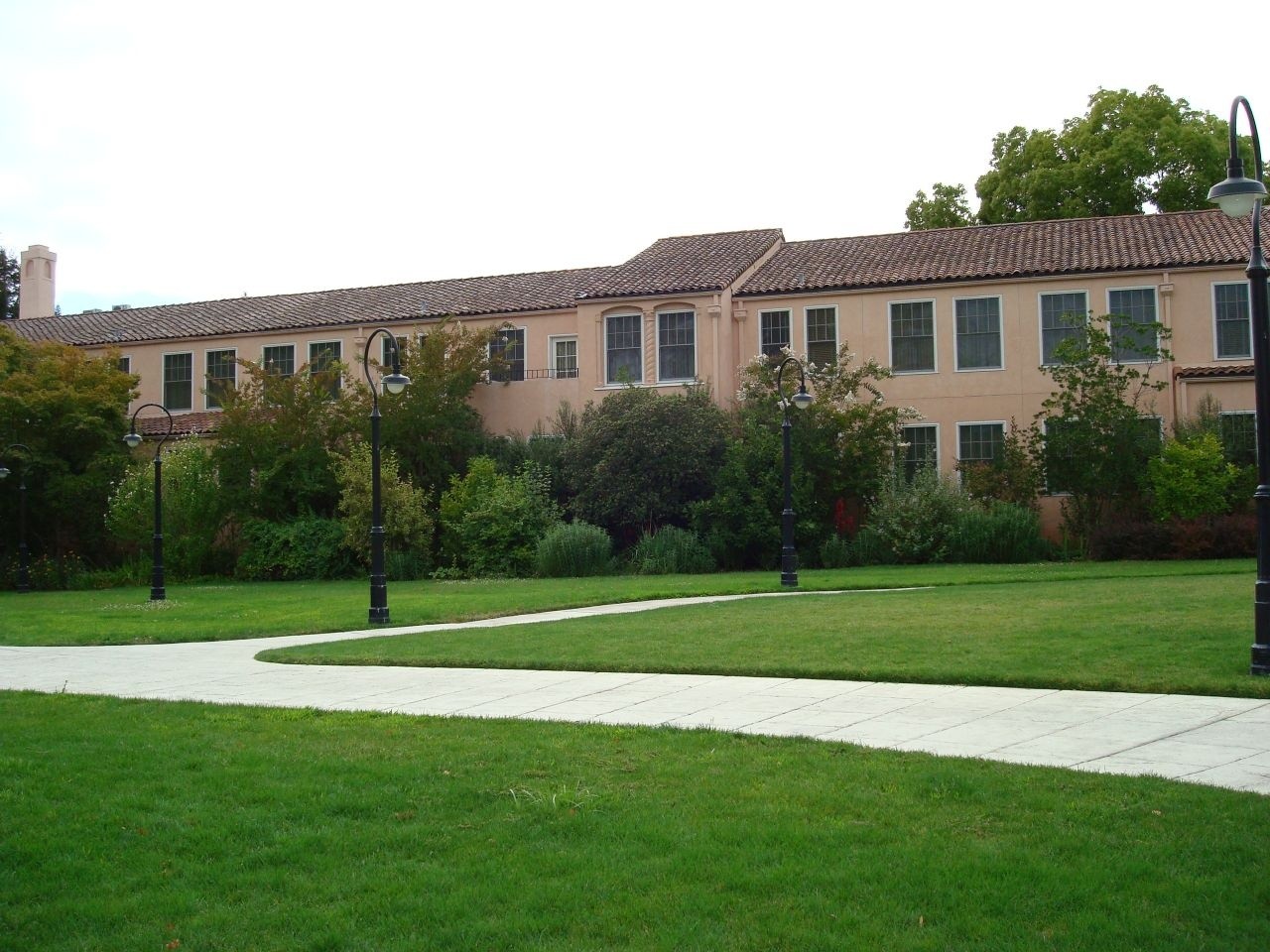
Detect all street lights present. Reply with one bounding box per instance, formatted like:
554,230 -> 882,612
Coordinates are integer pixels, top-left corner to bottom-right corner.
363,326 -> 414,625
1206,94 -> 1270,674
776,356 -> 815,589
122,402 -> 173,600
